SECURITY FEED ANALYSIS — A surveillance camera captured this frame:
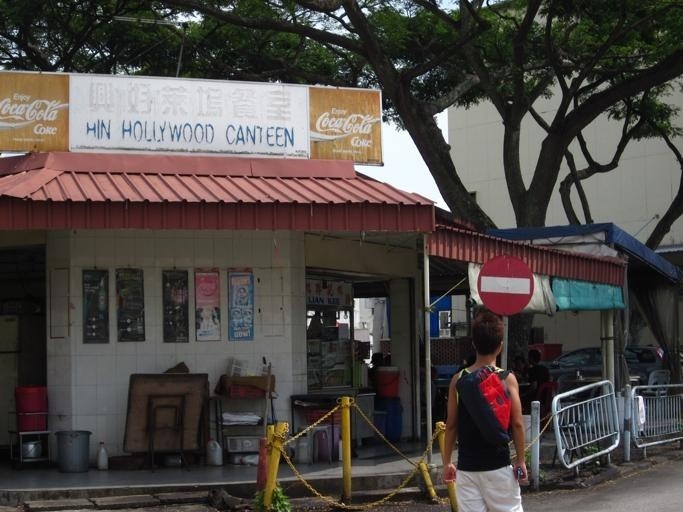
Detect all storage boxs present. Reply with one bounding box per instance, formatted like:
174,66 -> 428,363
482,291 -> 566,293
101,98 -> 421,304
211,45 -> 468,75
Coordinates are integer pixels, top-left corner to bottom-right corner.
214,374 -> 275,396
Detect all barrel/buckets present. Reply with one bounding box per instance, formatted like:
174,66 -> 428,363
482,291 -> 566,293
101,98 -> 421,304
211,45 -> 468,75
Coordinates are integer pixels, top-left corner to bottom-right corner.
522,415 -> 531,442
21,441 -> 42,457
374,397 -> 403,441
375,366 -> 400,398
54,430 -> 93,473
239,454 -> 258,466
14,385 -> 48,431
96,440 -> 108,470
206,438 -> 223,466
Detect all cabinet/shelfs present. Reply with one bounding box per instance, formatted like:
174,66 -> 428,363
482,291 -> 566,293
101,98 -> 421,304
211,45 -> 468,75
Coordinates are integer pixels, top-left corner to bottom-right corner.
209,396 -> 276,465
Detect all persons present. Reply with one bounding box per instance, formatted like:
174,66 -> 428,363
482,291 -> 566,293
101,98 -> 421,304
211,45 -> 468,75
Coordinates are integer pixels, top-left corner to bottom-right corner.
512,348 -> 550,413
443,313 -> 527,512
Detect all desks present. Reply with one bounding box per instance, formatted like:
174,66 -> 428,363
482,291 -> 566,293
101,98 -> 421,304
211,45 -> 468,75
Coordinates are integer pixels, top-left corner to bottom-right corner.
9,430 -> 50,466
123,373 -> 208,472
290,394 -> 355,463
351,393 -> 376,446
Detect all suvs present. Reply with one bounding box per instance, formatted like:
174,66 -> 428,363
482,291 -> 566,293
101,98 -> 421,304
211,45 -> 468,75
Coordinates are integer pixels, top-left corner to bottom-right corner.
539,343 -> 672,399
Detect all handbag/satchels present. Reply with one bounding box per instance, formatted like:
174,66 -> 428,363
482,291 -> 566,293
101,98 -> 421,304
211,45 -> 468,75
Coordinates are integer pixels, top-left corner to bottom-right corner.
456,366 -> 513,448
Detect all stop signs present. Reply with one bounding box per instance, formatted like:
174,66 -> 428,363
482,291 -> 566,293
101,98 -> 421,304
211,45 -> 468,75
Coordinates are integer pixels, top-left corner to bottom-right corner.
476,253 -> 533,315
